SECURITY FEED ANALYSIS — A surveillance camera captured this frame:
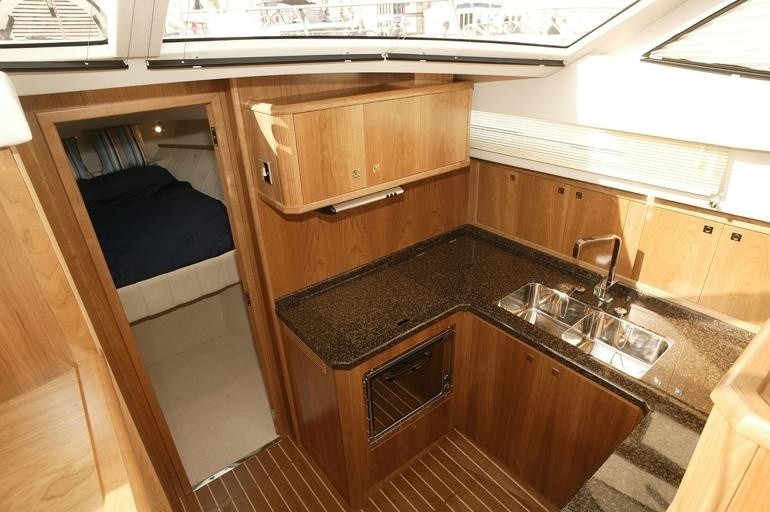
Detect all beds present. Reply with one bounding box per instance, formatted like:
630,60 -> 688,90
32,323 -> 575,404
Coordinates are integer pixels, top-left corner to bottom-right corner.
61,122 -> 242,325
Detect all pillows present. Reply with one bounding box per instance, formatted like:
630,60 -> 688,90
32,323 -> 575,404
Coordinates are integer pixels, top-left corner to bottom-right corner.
61,135 -> 95,181
84,123 -> 150,175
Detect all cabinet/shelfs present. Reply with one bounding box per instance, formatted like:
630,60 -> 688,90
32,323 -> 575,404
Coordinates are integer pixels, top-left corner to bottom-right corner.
1,148 -> 175,512
240,80 -> 475,215
272,312 -> 642,512
664,317 -> 770,512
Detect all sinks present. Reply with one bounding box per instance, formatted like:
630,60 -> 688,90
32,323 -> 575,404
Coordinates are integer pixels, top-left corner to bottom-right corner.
495,282 -> 584,338
562,310 -> 674,380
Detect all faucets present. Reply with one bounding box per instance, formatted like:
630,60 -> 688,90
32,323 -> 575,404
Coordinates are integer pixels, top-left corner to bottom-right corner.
573,234 -> 621,300
